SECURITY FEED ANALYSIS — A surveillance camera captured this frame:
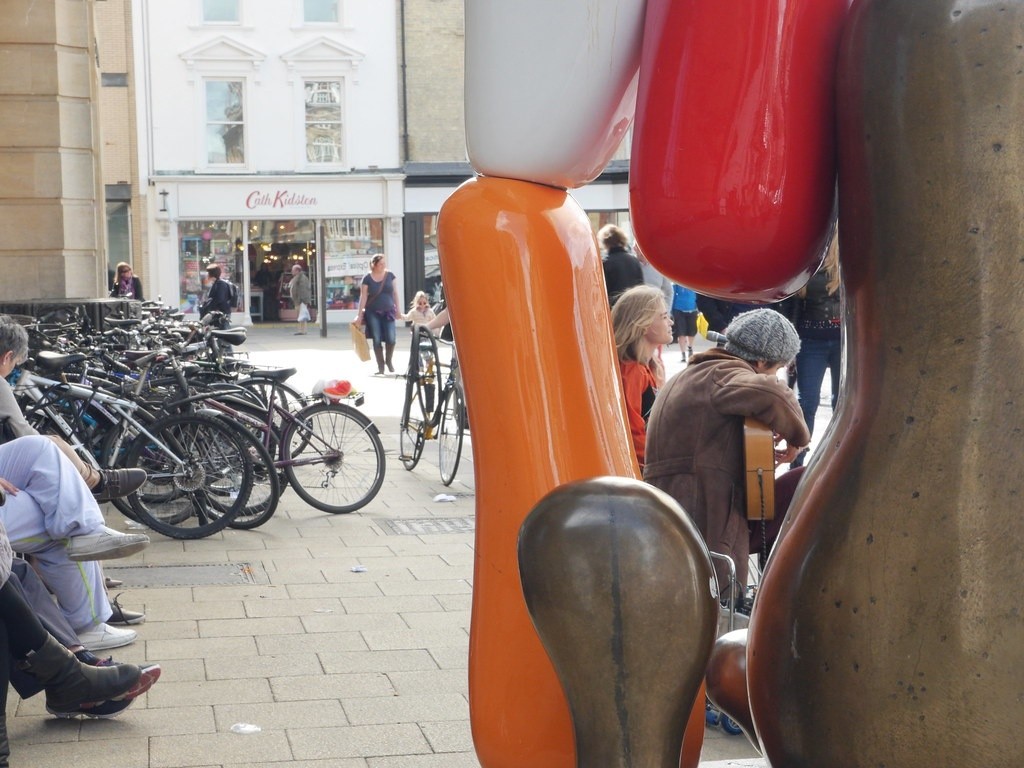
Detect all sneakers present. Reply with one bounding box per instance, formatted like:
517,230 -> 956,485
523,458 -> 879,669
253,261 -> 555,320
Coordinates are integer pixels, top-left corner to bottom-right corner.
69,528 -> 151,562
94,468 -> 147,501
76,622 -> 137,651
107,591 -> 146,625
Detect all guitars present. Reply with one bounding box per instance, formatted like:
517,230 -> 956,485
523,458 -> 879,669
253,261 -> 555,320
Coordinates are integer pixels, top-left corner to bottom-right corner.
742,416 -> 776,521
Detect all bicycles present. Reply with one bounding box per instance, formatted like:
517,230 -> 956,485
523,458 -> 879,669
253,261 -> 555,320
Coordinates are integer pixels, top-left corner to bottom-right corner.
9,296 -> 387,540
398,320 -> 467,488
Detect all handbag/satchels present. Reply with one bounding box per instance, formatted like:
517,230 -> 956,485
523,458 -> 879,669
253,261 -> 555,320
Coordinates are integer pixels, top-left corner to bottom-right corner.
352,323 -> 370,361
360,308 -> 367,325
696,312 -> 709,338
297,303 -> 311,322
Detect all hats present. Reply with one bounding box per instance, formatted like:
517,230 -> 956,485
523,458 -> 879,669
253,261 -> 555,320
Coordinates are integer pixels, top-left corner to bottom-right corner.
724,308 -> 801,362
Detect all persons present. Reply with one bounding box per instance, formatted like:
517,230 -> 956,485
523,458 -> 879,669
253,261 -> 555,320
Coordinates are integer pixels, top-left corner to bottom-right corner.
197,264 -> 235,373
352,254 -> 402,375
395,291 -> 442,376
0,518 -> 161,768
633,240 -> 798,392
598,223 -> 645,299
0,434 -> 153,652
642,306 -> 812,618
791,218 -> 841,470
0,314 -> 151,625
425,307 -> 450,329
288,264 -> 312,335
109,262 -> 145,302
610,284 -> 674,472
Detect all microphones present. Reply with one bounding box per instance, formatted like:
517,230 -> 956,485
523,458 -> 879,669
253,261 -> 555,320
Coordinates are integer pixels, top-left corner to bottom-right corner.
705,331 -> 728,345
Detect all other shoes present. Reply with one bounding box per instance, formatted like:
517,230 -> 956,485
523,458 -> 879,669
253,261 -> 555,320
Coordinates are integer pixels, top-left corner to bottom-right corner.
105,578 -> 122,586
295,332 -> 305,336
688,349 -> 693,356
22,632 -> 163,720
682,357 -> 686,362
456,407 -> 469,429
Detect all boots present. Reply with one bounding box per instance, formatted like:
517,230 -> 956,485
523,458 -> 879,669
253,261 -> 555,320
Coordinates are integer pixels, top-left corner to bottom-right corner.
0,713 -> 11,767
375,346 -> 384,374
386,344 -> 395,372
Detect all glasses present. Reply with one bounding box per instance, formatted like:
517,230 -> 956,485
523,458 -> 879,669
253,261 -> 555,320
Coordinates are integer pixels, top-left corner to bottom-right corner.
122,268 -> 131,273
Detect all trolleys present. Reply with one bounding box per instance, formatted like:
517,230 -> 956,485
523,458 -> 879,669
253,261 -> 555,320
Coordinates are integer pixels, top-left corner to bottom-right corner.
705,551 -> 742,735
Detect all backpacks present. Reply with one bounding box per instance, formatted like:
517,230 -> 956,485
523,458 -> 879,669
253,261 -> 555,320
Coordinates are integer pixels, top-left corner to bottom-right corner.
222,279 -> 240,308
